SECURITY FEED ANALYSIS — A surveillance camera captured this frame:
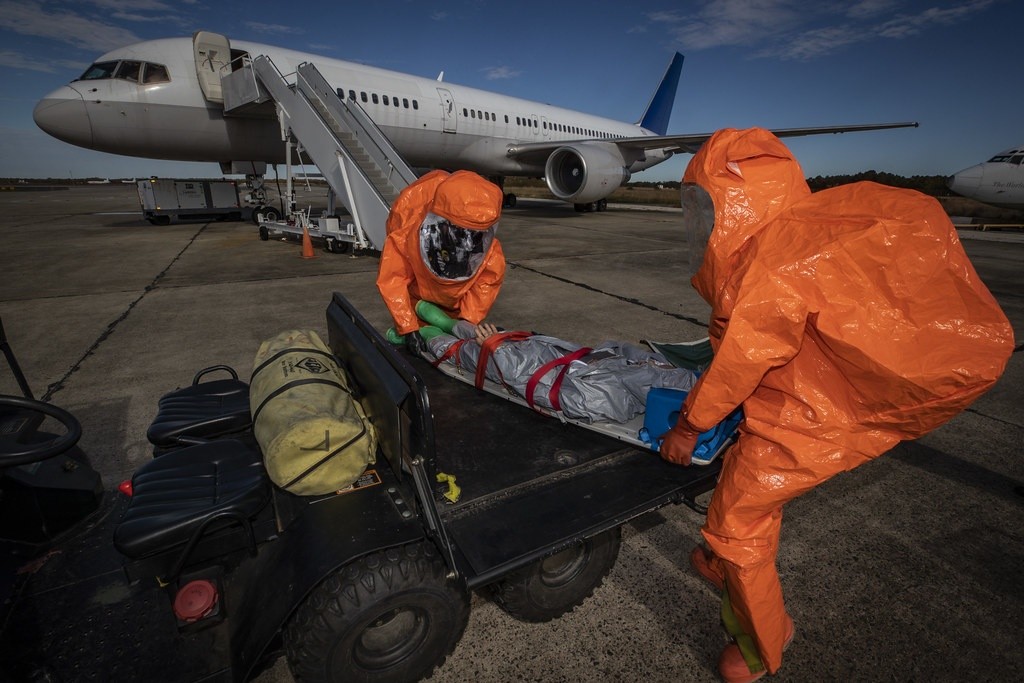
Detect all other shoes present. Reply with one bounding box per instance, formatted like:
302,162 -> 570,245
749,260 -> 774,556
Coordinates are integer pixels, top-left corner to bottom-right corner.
689,546 -> 726,592
718,611 -> 795,683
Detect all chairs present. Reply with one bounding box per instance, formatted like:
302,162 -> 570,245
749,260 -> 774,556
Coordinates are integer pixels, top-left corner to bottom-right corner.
113,363 -> 278,582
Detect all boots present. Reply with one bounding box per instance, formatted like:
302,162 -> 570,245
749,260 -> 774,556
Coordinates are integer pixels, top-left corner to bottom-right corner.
415,300 -> 458,334
386,325 -> 443,344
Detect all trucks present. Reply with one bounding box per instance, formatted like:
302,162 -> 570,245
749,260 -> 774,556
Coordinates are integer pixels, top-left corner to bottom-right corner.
1,291 -> 726,683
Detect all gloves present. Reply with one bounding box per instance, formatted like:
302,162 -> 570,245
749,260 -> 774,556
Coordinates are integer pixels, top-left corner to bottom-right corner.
405,330 -> 427,359
659,411 -> 700,466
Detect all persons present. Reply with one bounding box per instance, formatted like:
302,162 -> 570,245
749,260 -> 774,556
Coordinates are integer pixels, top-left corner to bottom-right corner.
658,128 -> 1015,683
377,170 -> 505,359
387,300 -> 698,421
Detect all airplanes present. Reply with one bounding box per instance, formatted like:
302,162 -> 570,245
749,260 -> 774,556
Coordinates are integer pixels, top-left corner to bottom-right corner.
31,28 -> 920,257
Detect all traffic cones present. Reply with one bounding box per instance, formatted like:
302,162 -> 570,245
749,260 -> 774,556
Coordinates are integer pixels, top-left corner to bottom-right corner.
296,225 -> 321,260
